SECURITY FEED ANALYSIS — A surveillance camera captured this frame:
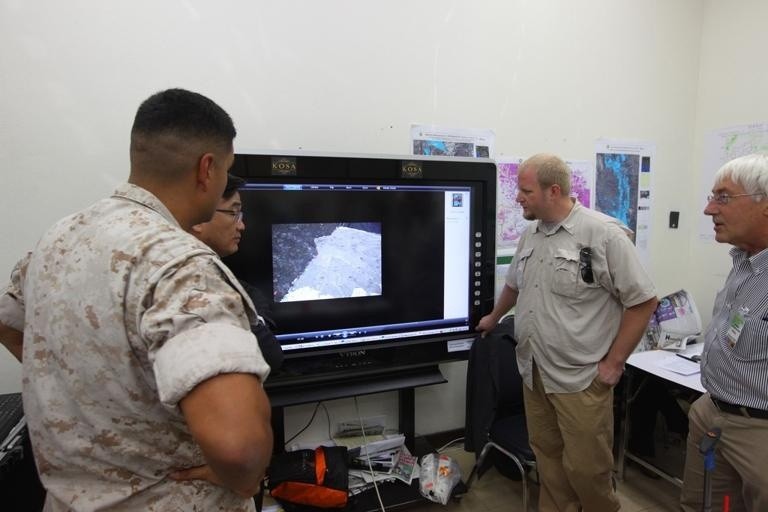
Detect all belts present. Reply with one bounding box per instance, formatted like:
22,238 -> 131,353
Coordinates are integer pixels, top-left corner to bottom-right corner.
710,394 -> 768,419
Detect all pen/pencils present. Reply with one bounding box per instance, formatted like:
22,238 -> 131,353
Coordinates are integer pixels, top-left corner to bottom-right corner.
676,353 -> 697,364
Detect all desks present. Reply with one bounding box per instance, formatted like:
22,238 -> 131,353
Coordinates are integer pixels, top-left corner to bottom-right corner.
616,341 -> 707,512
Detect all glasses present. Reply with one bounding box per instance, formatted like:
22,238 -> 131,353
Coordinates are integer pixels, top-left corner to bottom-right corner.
216,210 -> 243,224
708,193 -> 765,205
580,248 -> 593,283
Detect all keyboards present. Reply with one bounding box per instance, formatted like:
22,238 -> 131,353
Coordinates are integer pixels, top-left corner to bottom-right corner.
0,392 -> 24,446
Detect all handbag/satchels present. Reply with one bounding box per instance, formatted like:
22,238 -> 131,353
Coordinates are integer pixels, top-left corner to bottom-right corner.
268,444 -> 348,509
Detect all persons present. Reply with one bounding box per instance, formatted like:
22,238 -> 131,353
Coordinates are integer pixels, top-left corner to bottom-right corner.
677,152 -> 768,511
188,171 -> 248,264
474,149 -> 659,511
1,84 -> 280,509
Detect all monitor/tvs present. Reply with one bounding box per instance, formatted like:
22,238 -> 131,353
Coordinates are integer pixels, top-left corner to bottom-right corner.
224,154 -> 497,390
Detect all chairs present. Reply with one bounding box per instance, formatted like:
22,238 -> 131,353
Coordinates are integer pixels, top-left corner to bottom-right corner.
475,314 -> 538,512
